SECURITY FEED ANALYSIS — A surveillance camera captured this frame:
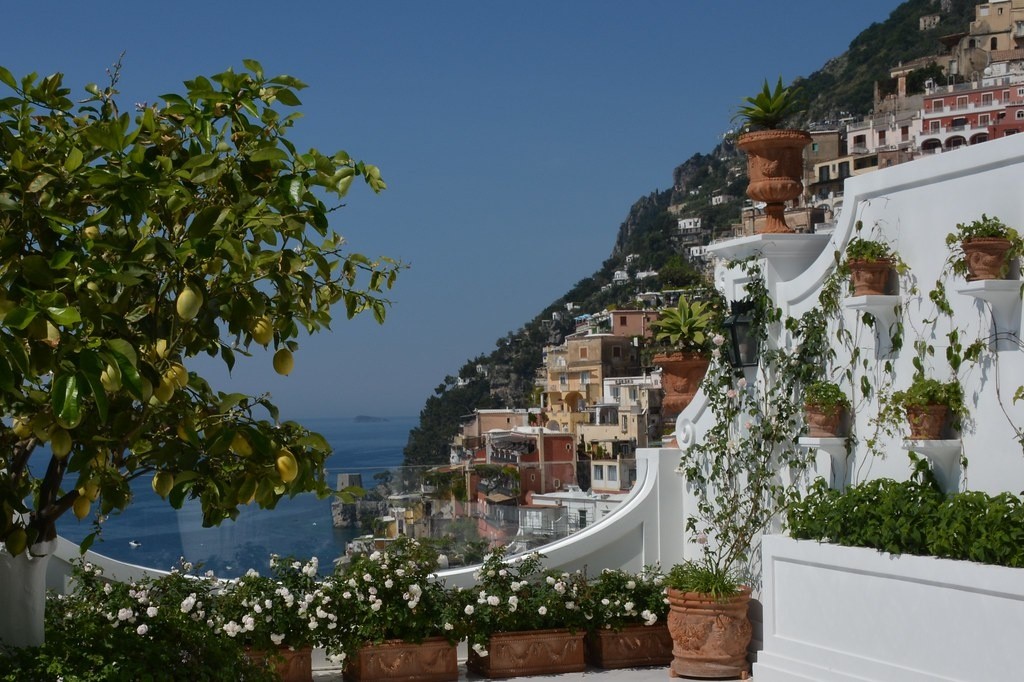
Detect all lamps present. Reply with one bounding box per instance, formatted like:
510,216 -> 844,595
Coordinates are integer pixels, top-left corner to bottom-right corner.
723,311 -> 759,367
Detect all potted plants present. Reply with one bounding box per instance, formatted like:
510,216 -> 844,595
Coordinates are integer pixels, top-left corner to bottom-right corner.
945,213 -> 1024,280
648,296 -> 720,424
833,220 -> 906,294
667,559 -> 759,679
725,74 -> 814,231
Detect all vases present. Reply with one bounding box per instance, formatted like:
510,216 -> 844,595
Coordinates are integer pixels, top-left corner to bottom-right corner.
466,626 -> 588,679
342,634 -> 461,681
594,620 -> 674,667
806,403 -> 846,437
904,403 -> 949,439
239,638 -> 314,681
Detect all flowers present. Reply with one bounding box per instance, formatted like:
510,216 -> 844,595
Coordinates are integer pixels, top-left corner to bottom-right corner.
801,379 -> 854,410
881,375 -> 971,430
43,531 -> 677,682
